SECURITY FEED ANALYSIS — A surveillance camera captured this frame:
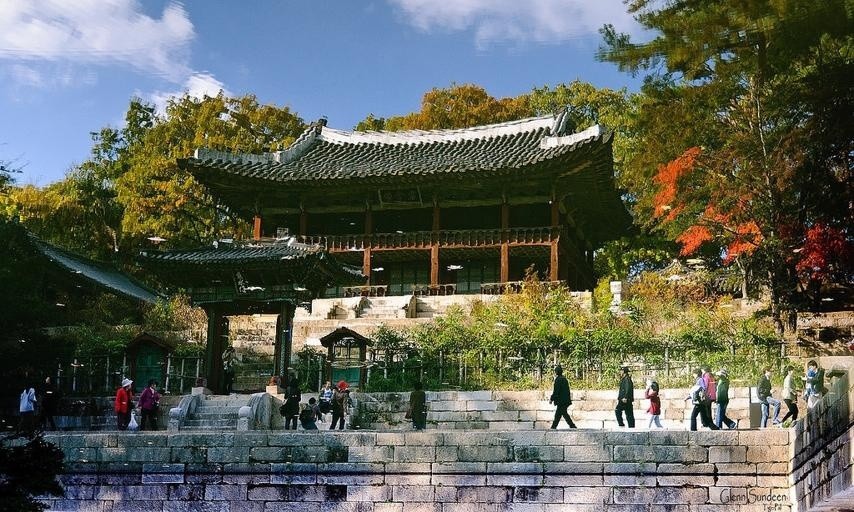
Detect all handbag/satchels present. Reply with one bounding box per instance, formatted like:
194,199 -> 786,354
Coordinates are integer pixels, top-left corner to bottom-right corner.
406,408 -> 412,418
300,405 -> 312,422
280,404 -> 287,417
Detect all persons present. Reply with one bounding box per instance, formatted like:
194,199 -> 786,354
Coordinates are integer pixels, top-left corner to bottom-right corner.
38,377 -> 57,433
408,383 -> 426,434
646,382 -> 663,427
18,376 -> 38,442
615,368 -> 635,427
114,379 -> 162,430
756,359 -> 823,428
261,376 -> 352,429
550,365 -> 576,428
683,364 -> 738,430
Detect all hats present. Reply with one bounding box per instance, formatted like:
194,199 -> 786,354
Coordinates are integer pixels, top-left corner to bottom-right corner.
148,380 -> 158,387
337,381 -> 348,389
121,379 -> 133,387
